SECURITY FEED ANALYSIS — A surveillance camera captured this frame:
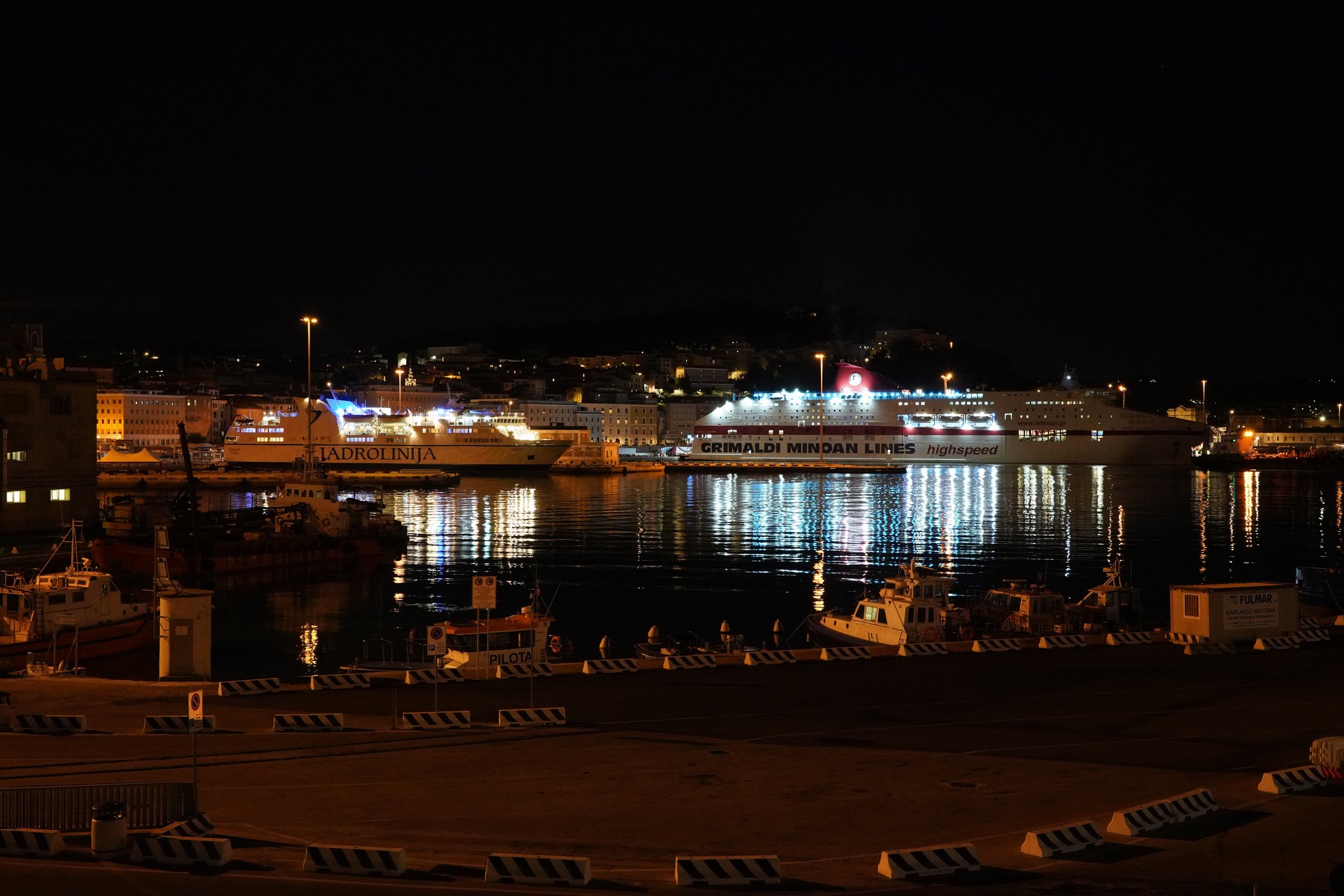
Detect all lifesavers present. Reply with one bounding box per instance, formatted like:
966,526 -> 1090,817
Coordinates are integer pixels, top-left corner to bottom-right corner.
551,636 -> 562,653
961,626 -> 974,641
923,626 -> 937,642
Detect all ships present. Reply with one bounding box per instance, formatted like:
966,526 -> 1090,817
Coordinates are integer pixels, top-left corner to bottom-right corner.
223,390 -> 571,475
692,389 -> 1211,465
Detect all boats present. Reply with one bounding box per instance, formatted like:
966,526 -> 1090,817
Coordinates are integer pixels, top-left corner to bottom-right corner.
633,638 -> 759,656
1191,434 -> 1246,469
402,579 -> 560,670
808,560 -> 1149,647
92,477 -> 415,597
0,519 -> 156,675
